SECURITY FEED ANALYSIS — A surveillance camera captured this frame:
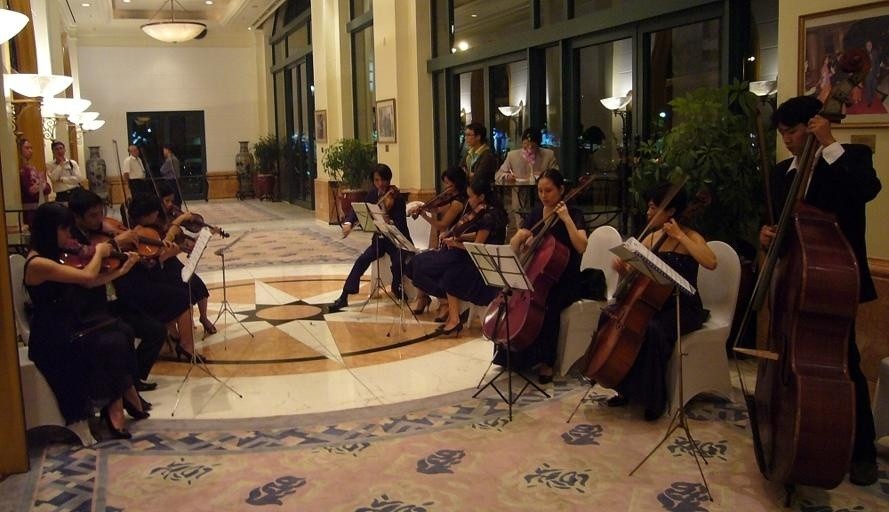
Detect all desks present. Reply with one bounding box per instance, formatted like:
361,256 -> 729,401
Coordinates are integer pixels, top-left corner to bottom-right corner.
494,179 -> 540,232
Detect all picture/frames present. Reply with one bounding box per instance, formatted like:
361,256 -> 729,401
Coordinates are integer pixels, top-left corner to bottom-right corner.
314,109 -> 328,143
796,0 -> 888,130
376,98 -> 397,143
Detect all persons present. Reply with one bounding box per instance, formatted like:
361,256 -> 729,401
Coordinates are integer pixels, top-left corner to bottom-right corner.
495,127 -> 559,228
494,168 -> 605,385
810,40 -> 880,108
607,182 -> 717,421
19,139 -> 51,232
406,179 -> 507,339
461,122 -> 504,209
47,142 -> 83,202
160,145 -> 182,208
759,95 -> 881,487
332,163 -> 414,308
124,144 -> 145,198
412,167 -> 465,322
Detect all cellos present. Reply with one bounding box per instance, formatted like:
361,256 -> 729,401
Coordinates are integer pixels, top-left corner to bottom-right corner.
578,187 -> 712,389
481,175 -> 595,353
732,47 -> 871,491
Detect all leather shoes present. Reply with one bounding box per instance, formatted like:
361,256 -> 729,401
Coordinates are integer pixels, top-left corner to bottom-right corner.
390,283 -> 407,301
609,384 -> 638,407
329,295 -> 348,311
645,382 -> 665,422
538,363 -> 554,385
854,439 -> 875,482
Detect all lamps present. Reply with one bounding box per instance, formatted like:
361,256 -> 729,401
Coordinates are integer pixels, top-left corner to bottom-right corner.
599,96 -> 631,155
139,0 -> 207,44
0,8 -> 106,132
498,106 -> 521,146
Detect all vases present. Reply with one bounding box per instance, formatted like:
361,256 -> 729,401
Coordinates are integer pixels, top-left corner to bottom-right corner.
85,146 -> 106,198
235,141 -> 254,190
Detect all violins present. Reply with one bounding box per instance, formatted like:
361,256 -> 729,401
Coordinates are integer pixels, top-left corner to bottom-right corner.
58,205 -> 231,274
376,188 -> 488,249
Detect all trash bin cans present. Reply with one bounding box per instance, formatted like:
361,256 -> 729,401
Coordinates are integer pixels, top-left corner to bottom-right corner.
186,159 -> 203,193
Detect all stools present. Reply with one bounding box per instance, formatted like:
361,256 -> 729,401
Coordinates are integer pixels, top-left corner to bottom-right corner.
17,345 -> 98,448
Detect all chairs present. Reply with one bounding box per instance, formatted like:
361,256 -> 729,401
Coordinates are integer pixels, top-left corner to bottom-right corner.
665,239 -> 742,418
554,224 -> 625,378
370,201 -> 432,301
8,253 -> 31,346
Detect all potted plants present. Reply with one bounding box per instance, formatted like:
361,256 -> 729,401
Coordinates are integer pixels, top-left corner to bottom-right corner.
251,134 -> 278,193
281,132 -> 307,192
319,137 -> 377,216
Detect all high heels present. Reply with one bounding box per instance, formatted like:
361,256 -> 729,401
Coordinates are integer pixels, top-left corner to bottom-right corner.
412,295 -> 463,336
99,382 -> 156,436
167,317 -> 217,359
